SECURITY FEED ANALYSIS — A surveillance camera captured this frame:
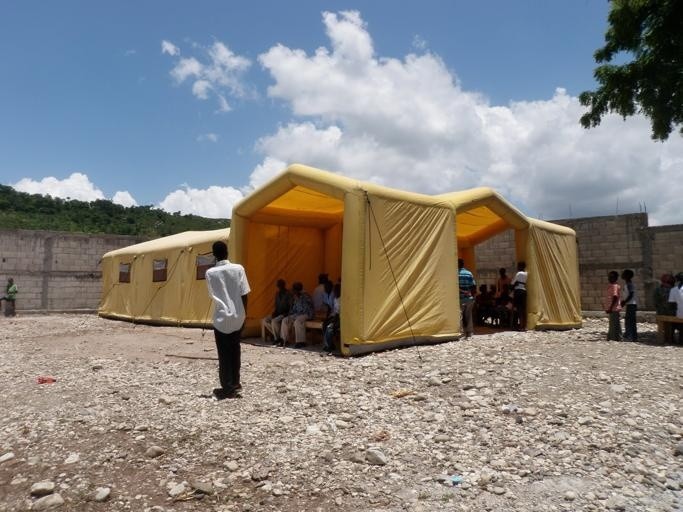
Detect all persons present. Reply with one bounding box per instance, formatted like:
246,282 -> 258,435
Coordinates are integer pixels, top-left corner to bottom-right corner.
475,283 -> 520,328
204,241 -> 252,397
665,272 -> 683,347
496,268 -> 511,291
275,280 -> 314,348
6,278 -> 18,316
506,261 -> 528,331
604,271 -> 624,341
619,269 -> 639,342
320,283 -> 341,356
311,273 -> 329,312
320,279 -> 334,310
652,274 -> 676,346
262,278 -> 293,345
456,258 -> 476,337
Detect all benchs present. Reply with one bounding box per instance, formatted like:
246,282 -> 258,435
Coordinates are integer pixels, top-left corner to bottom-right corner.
262,310 -> 327,342
656,315 -> 683,346
